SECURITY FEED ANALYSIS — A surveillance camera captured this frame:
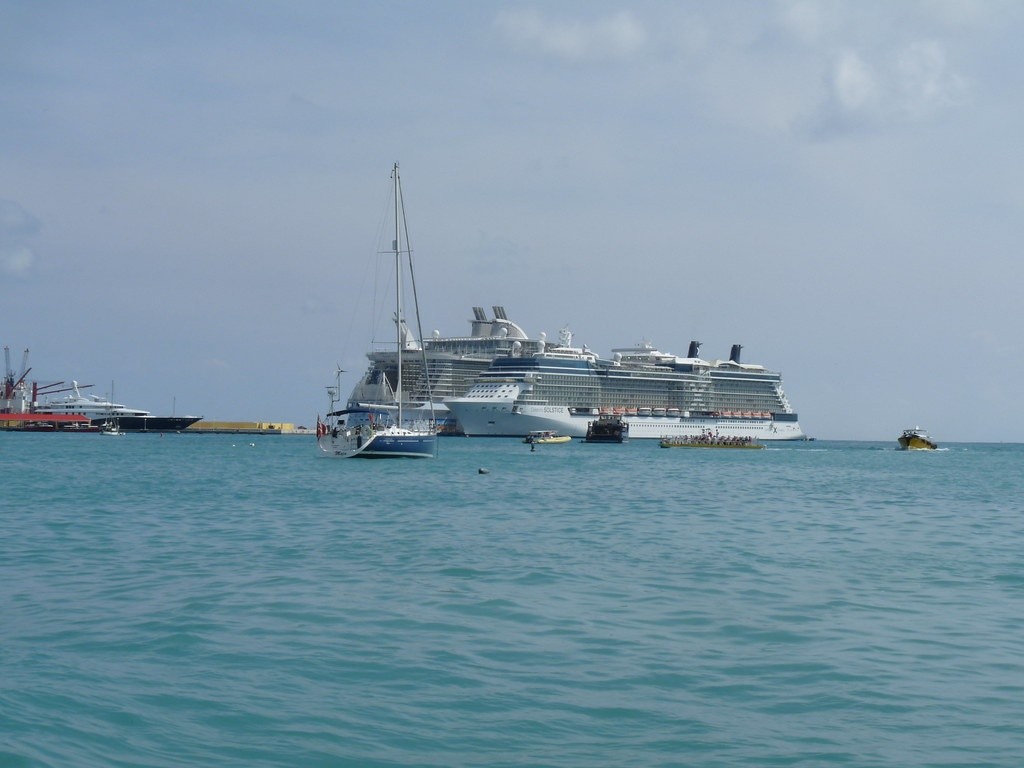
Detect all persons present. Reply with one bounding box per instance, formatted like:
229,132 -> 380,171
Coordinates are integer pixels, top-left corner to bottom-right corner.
333,422 -> 376,438
675,431 -> 752,446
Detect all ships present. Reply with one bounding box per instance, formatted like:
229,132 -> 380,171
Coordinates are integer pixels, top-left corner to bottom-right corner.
342,306 -> 669,429
442,345 -> 806,442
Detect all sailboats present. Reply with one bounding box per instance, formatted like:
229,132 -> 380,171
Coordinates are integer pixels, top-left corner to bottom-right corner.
98,381 -> 123,435
316,159 -> 439,457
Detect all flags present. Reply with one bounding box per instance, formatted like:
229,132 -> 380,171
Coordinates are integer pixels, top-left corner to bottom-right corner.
316,414 -> 322,441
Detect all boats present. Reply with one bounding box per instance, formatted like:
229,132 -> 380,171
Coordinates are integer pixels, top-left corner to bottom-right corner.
658,431 -> 763,448
584,411 -> 630,443
652,407 -> 667,416
523,429 -> 572,444
897,426 -> 940,451
710,411 -> 771,420
0,366 -> 93,433
625,408 -> 637,415
600,407 -> 612,414
612,407 -> 625,415
668,409 -> 680,416
23,380 -> 202,430
638,407 -> 652,416
